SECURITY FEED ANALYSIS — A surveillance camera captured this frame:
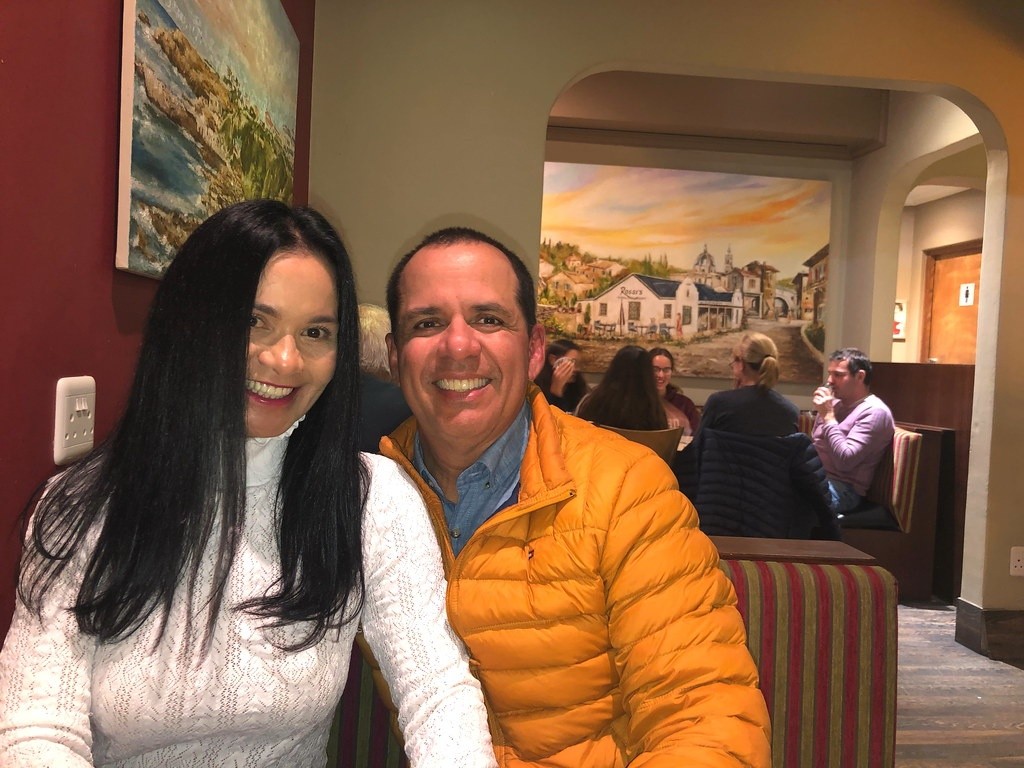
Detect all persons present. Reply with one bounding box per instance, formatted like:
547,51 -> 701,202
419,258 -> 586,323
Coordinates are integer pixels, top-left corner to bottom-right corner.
537,336 -> 701,506
0,199 -> 500,768
806,348 -> 898,512
672,331 -> 846,540
355,225 -> 773,768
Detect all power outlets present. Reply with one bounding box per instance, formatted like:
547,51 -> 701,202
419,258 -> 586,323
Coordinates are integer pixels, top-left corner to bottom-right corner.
1010,546 -> 1024,576
53,375 -> 94,464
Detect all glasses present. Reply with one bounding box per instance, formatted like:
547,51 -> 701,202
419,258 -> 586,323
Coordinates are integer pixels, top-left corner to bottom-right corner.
728,356 -> 742,370
653,366 -> 674,374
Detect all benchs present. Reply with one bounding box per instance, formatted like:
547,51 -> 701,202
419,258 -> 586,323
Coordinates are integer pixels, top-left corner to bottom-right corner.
327,406 -> 960,768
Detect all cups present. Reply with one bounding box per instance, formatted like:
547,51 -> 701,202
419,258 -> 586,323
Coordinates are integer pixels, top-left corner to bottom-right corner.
818,382 -> 834,395
